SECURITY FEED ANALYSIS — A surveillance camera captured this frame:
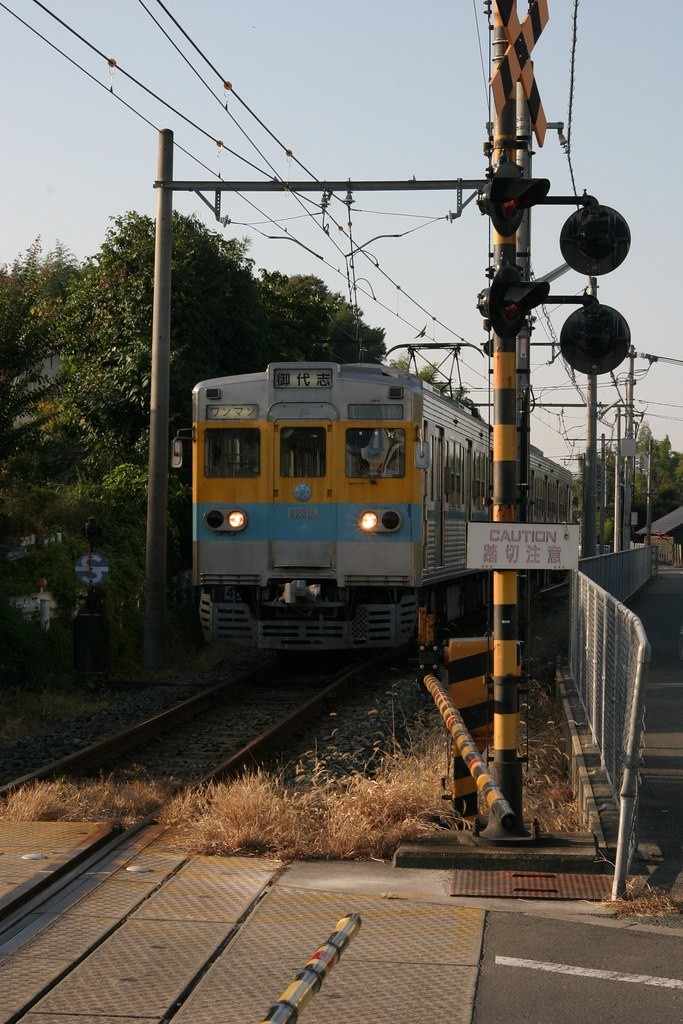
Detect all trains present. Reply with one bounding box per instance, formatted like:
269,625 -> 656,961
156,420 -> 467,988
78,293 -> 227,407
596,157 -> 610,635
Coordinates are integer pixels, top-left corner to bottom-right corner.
170,362 -> 577,669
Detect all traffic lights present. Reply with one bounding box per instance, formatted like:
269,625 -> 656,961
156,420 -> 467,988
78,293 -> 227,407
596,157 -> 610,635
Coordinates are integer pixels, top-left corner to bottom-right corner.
478,161 -> 551,238
476,265 -> 550,341
